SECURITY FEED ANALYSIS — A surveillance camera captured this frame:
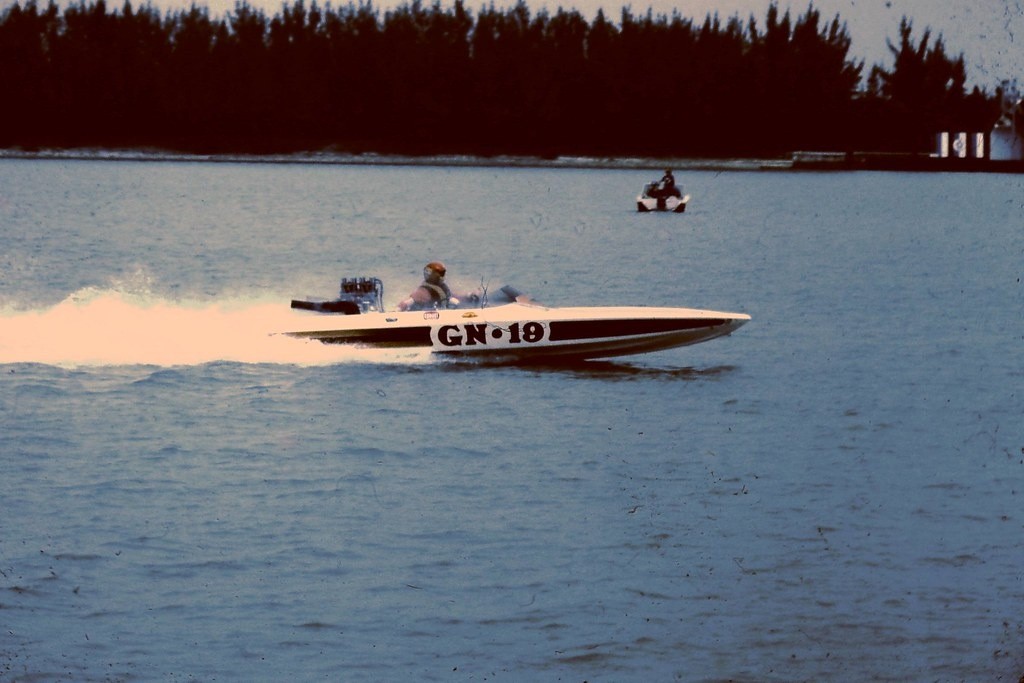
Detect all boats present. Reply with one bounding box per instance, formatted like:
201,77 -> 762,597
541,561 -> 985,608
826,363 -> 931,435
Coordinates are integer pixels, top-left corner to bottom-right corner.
270,307 -> 751,364
636,183 -> 685,211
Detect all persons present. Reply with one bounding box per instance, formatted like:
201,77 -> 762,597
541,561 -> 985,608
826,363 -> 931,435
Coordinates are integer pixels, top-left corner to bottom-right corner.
412,262 -> 453,311
648,182 -> 660,198
661,170 -> 674,189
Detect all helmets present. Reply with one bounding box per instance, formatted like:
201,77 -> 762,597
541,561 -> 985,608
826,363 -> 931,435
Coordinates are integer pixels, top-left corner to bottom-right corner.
423,262 -> 446,285
